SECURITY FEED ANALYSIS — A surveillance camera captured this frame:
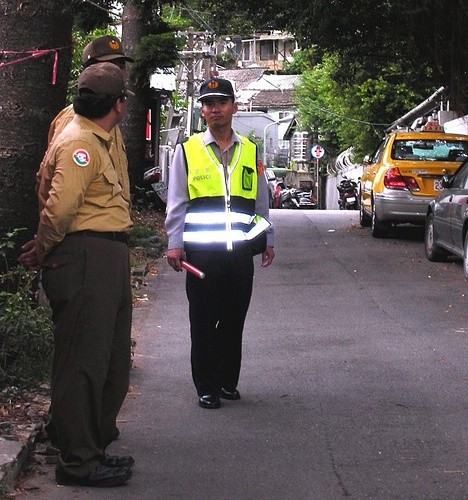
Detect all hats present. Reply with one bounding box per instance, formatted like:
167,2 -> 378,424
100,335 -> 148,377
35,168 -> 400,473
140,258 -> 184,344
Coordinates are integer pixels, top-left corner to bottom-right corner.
77,62 -> 136,98
82,36 -> 134,65
196,79 -> 234,101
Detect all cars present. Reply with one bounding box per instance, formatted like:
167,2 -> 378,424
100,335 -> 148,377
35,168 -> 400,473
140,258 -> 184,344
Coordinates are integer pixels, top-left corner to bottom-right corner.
356,121 -> 468,238
423,158 -> 468,282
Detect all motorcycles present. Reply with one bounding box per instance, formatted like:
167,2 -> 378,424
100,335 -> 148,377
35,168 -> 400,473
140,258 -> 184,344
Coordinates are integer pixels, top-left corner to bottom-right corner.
264,166 -> 317,209
336,171 -> 361,210
141,164 -> 168,215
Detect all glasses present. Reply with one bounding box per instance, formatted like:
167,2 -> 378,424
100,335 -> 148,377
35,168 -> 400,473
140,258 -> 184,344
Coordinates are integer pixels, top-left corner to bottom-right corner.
120,63 -> 129,71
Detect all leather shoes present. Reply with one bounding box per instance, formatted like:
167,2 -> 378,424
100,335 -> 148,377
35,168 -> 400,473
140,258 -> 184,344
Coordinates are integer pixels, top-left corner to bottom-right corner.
55,464 -> 133,487
198,393 -> 221,409
101,454 -> 134,466
218,385 -> 240,400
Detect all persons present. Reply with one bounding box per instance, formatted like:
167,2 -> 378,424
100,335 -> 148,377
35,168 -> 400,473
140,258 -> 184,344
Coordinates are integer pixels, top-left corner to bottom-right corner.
164,78 -> 275,409
20,62 -> 135,486
34,35 -> 130,463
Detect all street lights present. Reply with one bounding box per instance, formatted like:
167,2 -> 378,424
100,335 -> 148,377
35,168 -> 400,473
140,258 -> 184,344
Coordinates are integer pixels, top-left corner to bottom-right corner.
261,113 -> 296,169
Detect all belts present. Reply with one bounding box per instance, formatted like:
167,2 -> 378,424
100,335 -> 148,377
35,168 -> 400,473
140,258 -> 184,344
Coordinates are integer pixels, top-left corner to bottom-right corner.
79,230 -> 128,242
